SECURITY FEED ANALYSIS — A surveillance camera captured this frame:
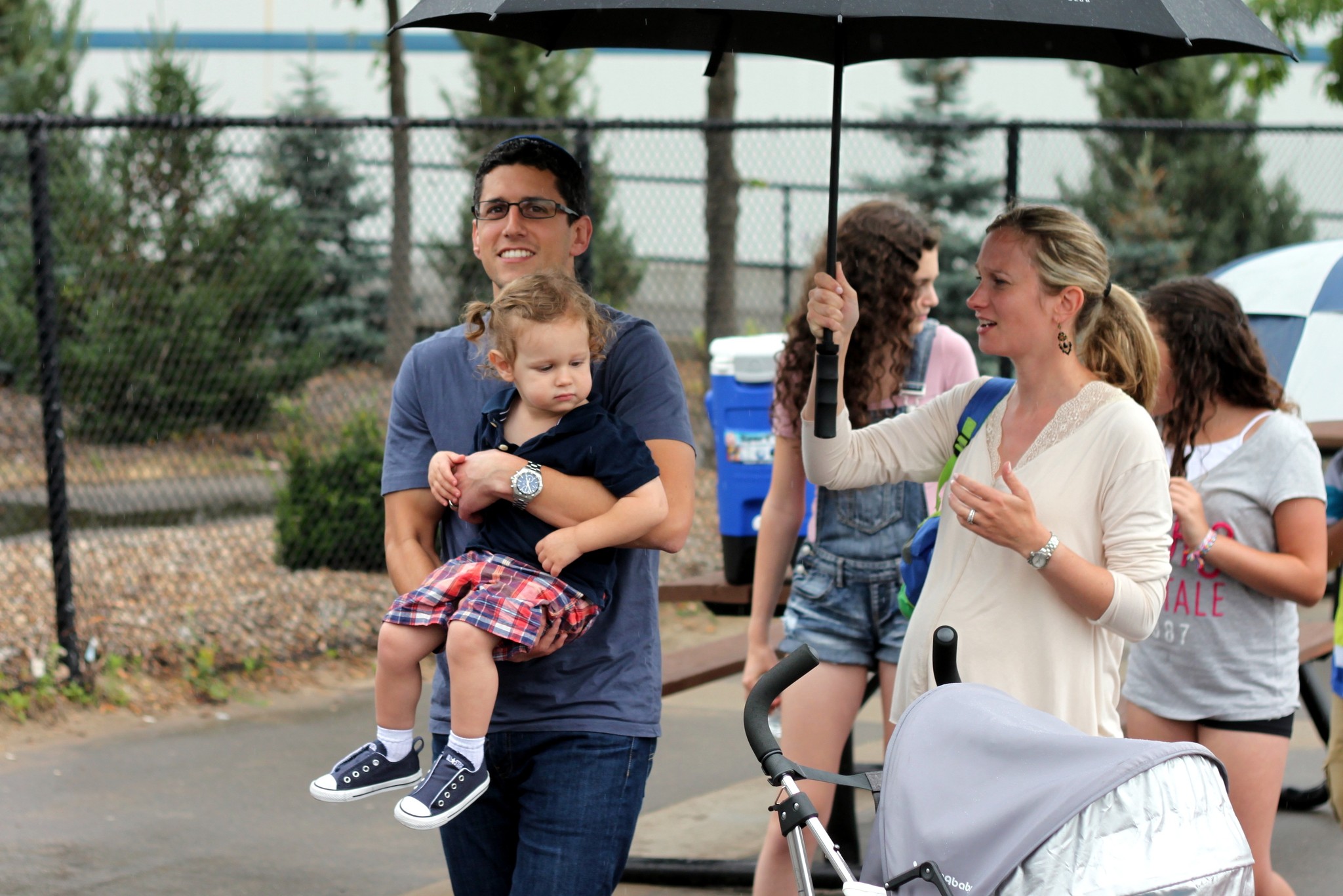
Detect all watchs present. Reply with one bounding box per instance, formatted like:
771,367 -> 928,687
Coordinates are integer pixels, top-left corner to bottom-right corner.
1026,531 -> 1060,571
510,461 -> 543,511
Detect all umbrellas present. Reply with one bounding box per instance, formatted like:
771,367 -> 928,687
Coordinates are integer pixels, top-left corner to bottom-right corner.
387,0 -> 1302,439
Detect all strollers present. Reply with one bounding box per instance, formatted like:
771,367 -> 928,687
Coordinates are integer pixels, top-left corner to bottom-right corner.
742,625 -> 1257,896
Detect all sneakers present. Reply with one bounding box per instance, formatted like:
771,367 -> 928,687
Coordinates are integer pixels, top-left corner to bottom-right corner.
393,744 -> 492,830
309,734 -> 426,803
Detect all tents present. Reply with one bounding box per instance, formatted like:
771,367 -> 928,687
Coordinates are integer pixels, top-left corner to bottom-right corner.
1209,239 -> 1343,423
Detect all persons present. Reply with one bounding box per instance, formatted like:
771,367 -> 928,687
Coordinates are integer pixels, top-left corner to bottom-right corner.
740,197 -> 980,895
801,205 -> 1174,738
1123,275 -> 1343,895
381,138 -> 696,896
308,270 -> 670,830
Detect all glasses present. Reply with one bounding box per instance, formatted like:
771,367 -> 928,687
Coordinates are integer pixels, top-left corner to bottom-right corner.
470,199 -> 582,221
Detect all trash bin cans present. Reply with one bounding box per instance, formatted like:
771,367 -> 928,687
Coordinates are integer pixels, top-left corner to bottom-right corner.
704,332 -> 814,587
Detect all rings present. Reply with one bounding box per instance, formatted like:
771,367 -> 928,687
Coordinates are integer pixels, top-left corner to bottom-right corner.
967,509 -> 975,524
449,499 -> 459,507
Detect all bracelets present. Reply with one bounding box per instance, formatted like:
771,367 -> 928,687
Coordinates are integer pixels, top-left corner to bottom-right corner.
1200,532 -> 1217,557
1183,528 -> 1213,569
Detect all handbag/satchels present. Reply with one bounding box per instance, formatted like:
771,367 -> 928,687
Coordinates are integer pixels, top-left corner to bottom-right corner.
894,376 -> 1016,617
